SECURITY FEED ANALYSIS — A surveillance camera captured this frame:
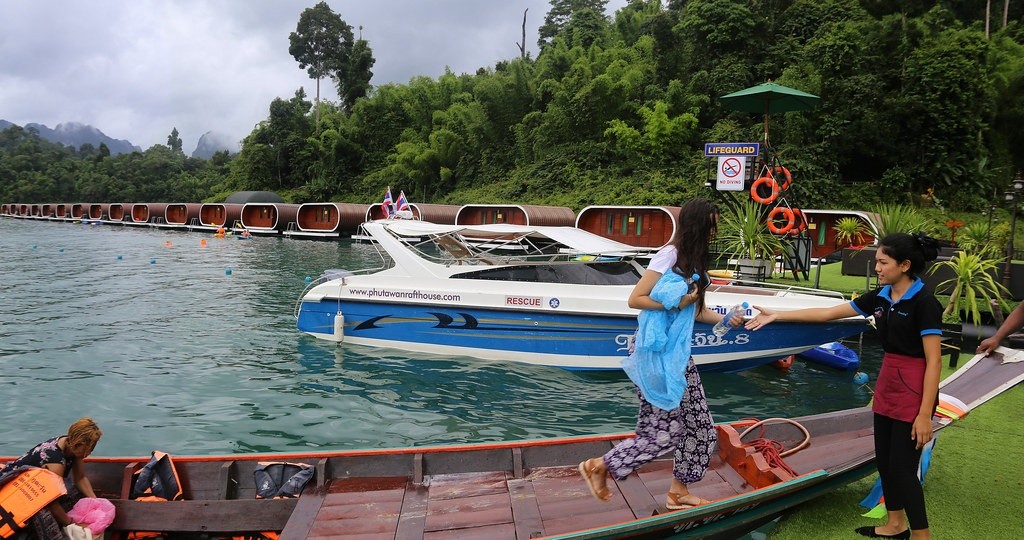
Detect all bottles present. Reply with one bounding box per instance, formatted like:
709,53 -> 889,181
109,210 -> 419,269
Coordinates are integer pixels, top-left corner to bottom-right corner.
712,302 -> 748,336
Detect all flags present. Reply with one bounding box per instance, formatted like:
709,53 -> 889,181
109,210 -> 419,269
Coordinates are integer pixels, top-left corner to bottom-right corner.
382,187 -> 410,216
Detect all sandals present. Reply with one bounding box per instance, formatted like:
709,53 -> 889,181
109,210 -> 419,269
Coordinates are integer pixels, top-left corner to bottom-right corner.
578,458 -> 611,503
666,491 -> 714,510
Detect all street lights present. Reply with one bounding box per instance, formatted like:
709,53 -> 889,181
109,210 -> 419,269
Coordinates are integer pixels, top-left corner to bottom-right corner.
982,199 -> 998,246
1001,177 -> 1024,298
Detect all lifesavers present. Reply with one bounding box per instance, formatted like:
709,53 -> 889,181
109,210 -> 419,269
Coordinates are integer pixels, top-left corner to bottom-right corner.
750,166 -> 792,205
767,207 -> 808,235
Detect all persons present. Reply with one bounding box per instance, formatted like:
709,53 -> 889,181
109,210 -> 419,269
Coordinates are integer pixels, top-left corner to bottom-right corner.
579,199 -> 744,509
749,233 -> 945,540
975,301 -> 1024,357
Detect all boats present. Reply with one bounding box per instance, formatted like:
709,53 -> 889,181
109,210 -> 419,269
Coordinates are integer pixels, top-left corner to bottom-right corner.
801,342 -> 860,371
0,340 -> 1023,540
294,203 -> 875,371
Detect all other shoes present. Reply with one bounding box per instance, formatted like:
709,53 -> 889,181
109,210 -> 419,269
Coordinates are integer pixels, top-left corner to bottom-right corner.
855,526 -> 910,540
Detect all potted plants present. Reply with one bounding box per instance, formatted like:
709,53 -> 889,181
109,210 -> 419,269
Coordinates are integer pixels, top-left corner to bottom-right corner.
714,200 -> 796,285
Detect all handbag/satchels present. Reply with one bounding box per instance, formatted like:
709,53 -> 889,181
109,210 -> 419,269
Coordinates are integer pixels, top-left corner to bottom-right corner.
63,523 -> 104,540
